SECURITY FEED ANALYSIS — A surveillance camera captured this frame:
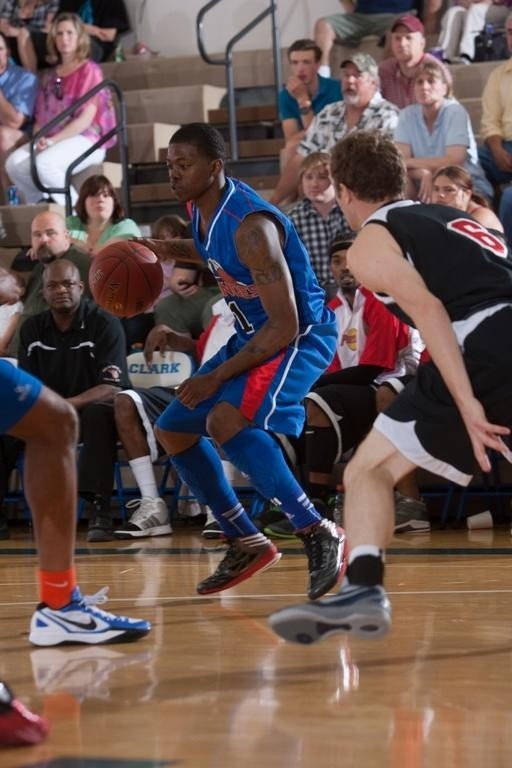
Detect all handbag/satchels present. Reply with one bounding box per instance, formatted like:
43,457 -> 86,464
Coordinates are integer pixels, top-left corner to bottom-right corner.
475,26 -> 509,60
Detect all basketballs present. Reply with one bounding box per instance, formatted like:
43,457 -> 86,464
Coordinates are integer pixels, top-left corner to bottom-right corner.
90,241 -> 163,318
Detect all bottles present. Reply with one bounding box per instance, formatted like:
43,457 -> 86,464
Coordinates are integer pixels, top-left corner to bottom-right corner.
333,479 -> 343,527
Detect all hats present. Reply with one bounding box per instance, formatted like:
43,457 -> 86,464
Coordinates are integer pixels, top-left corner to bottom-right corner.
341,50 -> 378,79
390,15 -> 424,37
330,234 -> 357,255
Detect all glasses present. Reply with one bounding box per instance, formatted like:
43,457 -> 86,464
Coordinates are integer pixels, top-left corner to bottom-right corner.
55,77 -> 65,100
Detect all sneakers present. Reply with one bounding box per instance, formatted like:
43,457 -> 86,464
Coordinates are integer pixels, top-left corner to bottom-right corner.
114,497 -> 173,540
87,496 -> 114,543
29,586 -> 151,648
252,502 -> 288,530
197,533 -> 282,595
293,518 -> 347,600
394,491 -> 432,534
270,585 -> 391,644
202,514 -> 225,537
264,498 -> 327,538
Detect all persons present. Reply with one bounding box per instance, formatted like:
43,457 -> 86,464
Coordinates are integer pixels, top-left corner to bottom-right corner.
1,360 -> 151,646
127,122 -> 346,603
1,0 -> 510,535
269,129 -> 512,644
